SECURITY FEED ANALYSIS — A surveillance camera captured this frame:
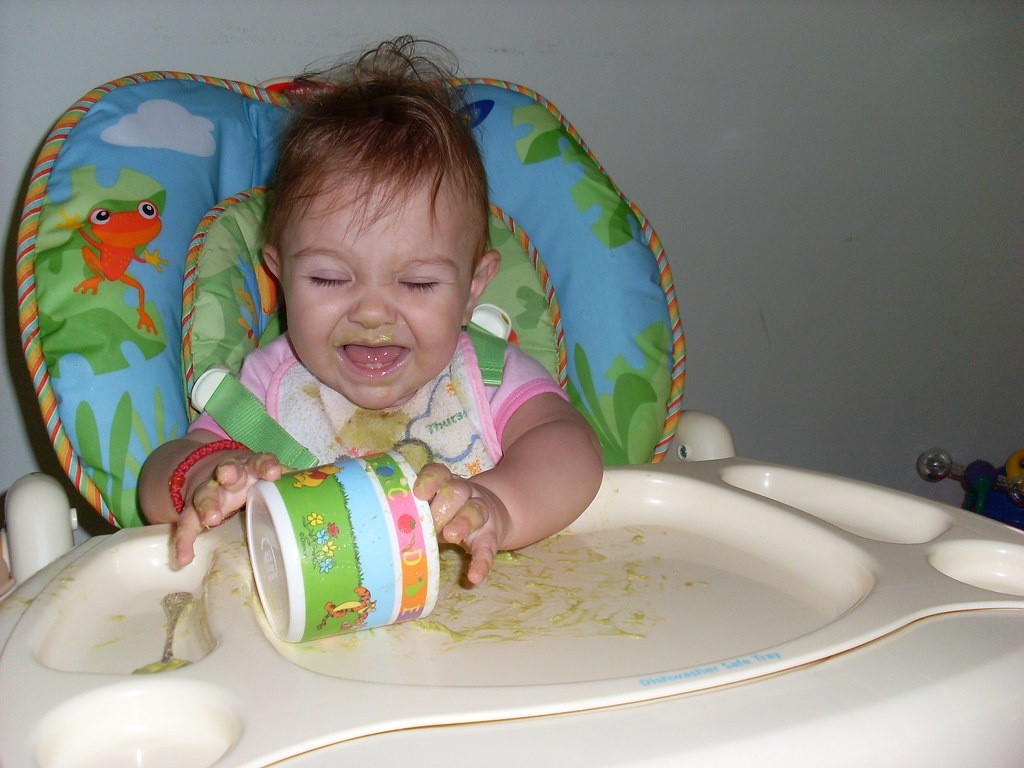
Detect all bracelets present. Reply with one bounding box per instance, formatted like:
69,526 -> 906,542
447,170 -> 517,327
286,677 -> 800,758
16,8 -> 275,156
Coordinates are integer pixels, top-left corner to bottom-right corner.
165,439 -> 255,517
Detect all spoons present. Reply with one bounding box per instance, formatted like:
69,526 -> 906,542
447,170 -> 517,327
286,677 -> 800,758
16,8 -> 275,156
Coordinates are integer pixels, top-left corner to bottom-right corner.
133,592 -> 194,673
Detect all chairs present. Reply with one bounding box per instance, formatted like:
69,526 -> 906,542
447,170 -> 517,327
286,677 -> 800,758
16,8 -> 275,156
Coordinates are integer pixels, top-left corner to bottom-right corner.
3,72 -> 1024,768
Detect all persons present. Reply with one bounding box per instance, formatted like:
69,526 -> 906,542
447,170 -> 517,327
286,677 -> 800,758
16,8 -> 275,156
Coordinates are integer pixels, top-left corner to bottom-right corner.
135,31 -> 606,592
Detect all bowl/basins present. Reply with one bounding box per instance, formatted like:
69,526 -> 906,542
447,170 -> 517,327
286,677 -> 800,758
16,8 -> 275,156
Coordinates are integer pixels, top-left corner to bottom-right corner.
244,451 -> 440,643
